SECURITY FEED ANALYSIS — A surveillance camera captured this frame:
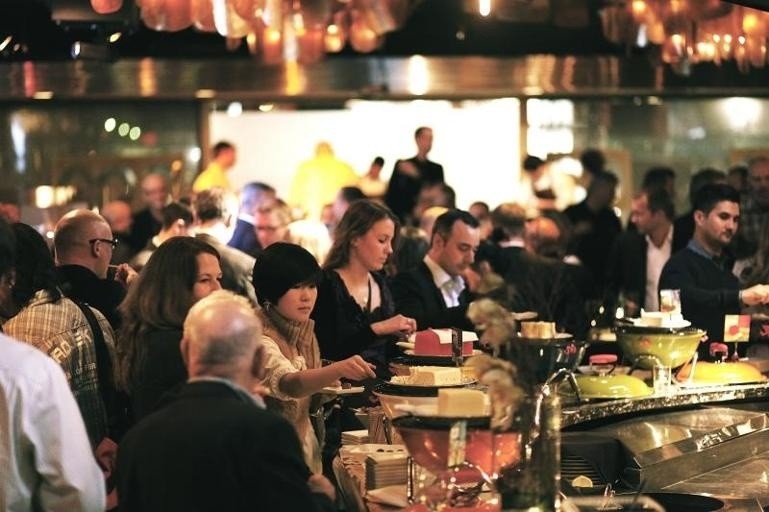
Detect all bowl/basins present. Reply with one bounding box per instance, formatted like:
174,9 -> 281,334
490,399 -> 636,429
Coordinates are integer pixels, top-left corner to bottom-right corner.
371,348 -> 525,485
615,327 -> 708,370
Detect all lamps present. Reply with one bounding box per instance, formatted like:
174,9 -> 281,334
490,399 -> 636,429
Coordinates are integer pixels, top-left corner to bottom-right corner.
597,1 -> 768,77
86,0 -> 412,67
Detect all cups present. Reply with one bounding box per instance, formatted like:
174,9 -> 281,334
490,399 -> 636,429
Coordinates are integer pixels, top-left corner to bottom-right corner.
519,333 -> 576,383
653,365 -> 673,398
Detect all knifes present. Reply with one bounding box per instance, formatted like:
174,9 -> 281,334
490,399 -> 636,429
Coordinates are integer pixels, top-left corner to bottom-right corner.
512,310 -> 539,320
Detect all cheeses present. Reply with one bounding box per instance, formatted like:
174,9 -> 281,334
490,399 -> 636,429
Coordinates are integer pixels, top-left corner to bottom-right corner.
639,308 -> 684,327
414,329 -> 474,356
521,320 -> 556,340
436,387 -> 492,417
389,364 -> 463,386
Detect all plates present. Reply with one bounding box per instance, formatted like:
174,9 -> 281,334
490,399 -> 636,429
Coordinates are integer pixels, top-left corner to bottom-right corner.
334,409 -> 412,493
577,341 -> 619,376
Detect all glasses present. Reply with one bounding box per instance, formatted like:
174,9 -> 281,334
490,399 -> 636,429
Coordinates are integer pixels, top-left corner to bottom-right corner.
88,238 -> 120,254
